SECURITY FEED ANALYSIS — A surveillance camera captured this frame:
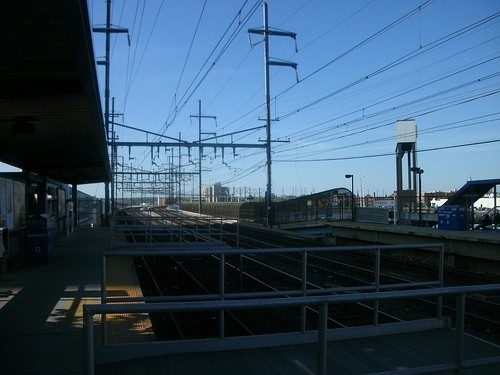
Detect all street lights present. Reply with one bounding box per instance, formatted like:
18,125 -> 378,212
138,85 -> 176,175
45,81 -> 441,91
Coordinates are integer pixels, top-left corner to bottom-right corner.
411,167 -> 424,223
345,175 -> 353,221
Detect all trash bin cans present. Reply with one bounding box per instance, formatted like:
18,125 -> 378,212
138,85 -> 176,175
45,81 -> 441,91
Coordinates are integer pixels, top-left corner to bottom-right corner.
23,214 -> 54,261
438,205 -> 469,231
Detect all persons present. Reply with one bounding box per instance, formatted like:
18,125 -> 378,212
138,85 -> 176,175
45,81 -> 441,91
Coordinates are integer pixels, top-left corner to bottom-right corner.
478,212 -> 500,232
